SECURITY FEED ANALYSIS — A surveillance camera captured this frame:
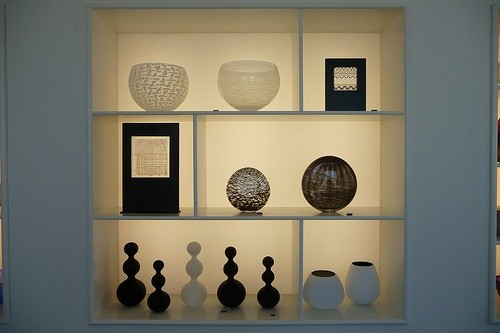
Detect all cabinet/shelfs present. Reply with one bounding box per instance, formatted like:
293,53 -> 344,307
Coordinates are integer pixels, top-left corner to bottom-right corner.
89,8 -> 406,325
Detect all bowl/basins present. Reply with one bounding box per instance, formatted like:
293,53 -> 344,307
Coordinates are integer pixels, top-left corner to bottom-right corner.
218,60 -> 281,110
129,63 -> 189,113
